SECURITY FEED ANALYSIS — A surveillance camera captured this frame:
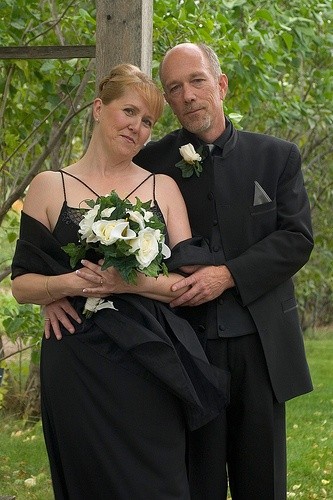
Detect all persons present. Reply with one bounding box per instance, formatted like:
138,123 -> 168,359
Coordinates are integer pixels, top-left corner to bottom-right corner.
132,42 -> 316,499
8,63 -> 215,500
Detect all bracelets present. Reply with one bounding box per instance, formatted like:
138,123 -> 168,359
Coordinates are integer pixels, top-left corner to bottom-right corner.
46,275 -> 56,303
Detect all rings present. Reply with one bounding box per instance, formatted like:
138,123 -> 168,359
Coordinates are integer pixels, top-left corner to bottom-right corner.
100,277 -> 103,286
44,318 -> 50,321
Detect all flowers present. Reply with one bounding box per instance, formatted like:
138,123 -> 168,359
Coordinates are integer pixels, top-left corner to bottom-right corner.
60,189 -> 172,320
173,143 -> 204,178
